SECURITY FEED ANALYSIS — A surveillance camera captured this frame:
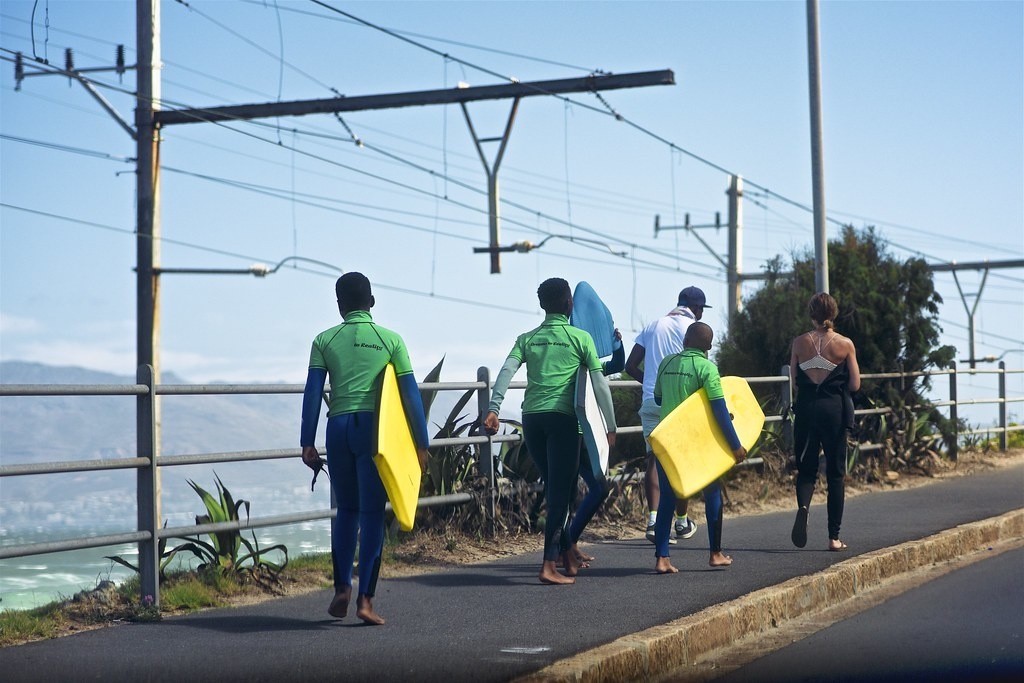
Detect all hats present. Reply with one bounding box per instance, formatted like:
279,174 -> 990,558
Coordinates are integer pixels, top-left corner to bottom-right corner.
677,286 -> 712,308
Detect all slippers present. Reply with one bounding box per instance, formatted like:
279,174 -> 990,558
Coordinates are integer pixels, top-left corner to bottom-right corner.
829,540 -> 848,551
791,507 -> 808,548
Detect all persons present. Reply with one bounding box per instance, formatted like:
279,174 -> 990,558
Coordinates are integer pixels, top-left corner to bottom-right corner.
654,322 -> 747,572
626,286 -> 713,544
484,277 -> 626,584
300,272 -> 429,624
791,292 -> 860,552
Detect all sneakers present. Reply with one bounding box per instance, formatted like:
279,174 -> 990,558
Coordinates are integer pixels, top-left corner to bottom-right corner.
646,521 -> 677,544
676,516 -> 697,539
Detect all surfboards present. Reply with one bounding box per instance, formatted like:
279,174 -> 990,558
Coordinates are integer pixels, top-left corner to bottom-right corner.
370,363 -> 423,531
574,363 -> 610,477
571,282 -> 621,358
649,376 -> 765,498
